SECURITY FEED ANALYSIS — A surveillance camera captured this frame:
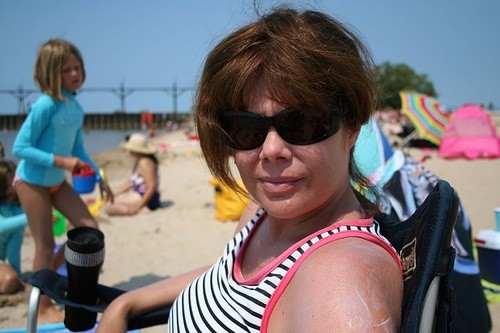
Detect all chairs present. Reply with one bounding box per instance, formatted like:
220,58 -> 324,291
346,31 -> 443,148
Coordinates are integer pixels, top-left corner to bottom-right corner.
23,179 -> 459,333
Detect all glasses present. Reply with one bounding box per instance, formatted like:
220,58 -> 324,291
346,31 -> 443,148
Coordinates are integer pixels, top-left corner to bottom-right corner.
222,100 -> 342,150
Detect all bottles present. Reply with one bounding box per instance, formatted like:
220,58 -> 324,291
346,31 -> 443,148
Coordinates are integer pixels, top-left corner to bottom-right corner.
64,225 -> 105,332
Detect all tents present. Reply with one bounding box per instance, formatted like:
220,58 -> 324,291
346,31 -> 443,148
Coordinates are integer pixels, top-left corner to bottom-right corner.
438,105 -> 500,159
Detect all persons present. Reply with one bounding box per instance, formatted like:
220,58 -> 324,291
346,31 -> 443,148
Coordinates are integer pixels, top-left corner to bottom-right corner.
12,38 -> 115,323
0,143 -> 20,294
106,133 -> 161,215
95,8 -> 404,333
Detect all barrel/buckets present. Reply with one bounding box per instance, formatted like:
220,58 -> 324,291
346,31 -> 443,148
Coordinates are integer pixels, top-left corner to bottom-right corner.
72,163 -> 96,193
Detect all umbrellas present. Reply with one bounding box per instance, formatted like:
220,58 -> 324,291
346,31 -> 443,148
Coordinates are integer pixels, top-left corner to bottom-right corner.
399,90 -> 450,146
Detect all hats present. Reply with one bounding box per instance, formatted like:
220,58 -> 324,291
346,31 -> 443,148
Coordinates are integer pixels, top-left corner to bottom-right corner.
121,132 -> 159,154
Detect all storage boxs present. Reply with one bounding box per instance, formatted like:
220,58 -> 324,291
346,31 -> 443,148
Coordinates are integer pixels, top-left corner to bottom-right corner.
473,230 -> 500,286
